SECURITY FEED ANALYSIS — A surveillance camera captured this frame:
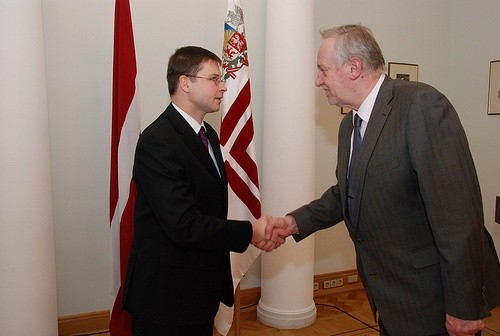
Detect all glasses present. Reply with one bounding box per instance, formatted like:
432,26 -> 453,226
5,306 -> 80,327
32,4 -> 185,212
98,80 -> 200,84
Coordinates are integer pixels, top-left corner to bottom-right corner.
180,74 -> 226,86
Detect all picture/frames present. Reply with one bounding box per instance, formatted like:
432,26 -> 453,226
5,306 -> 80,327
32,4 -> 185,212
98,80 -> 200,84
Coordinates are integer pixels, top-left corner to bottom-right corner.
487,60 -> 500,115
388,62 -> 418,82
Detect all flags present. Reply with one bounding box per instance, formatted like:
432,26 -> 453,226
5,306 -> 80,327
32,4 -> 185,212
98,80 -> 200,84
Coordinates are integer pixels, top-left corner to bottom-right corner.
110,0 -> 140,336
214,0 -> 264,336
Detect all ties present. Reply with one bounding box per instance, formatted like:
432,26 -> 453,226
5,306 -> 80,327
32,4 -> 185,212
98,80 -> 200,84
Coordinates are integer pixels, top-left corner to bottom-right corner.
198,126 -> 208,151
346,113 -> 363,225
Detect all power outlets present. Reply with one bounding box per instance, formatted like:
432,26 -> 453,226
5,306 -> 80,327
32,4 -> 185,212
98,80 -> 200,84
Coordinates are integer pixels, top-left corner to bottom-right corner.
331,280 -> 336,287
348,275 -> 358,282
324,281 -> 330,289
313,283 -> 319,290
338,278 -> 344,286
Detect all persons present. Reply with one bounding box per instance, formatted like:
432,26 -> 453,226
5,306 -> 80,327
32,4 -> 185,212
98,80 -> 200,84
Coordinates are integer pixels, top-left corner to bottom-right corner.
121,45 -> 296,336
257,24 -> 500,336
